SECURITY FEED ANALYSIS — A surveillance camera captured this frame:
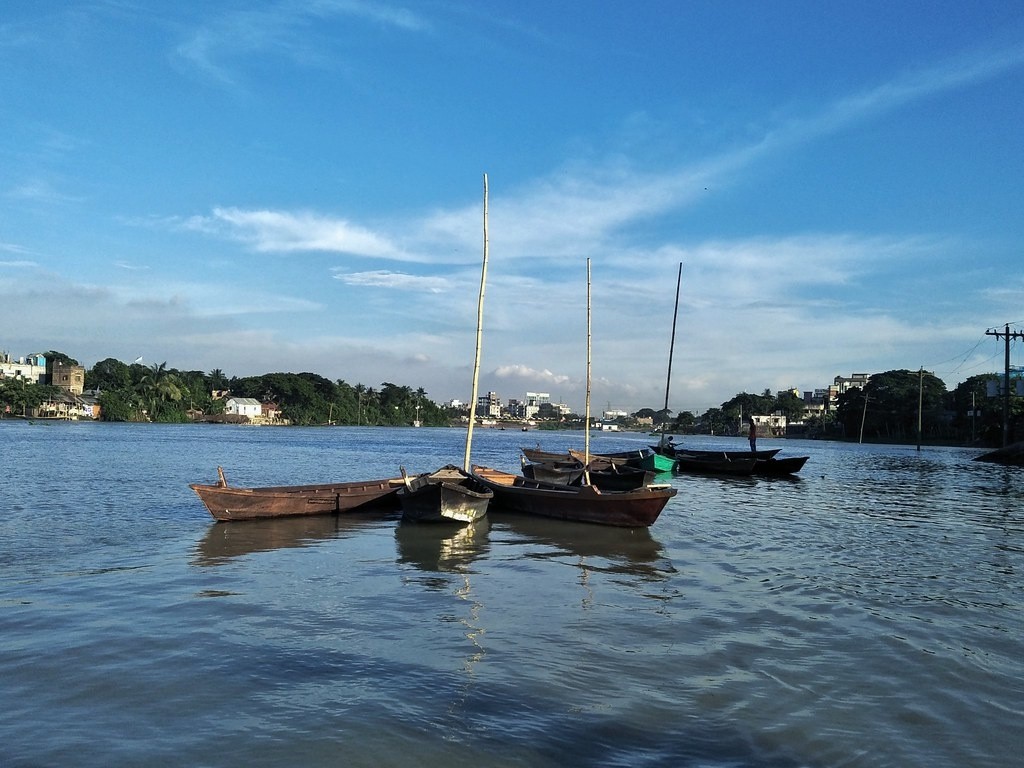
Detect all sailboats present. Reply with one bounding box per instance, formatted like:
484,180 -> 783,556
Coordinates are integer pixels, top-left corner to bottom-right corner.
521,257 -> 657,487
641,260 -> 682,472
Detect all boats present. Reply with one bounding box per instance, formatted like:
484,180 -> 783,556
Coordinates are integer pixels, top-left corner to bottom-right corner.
664,451 -> 811,475
468,464 -> 678,528
186,466 -> 431,522
396,461 -> 496,524
647,445 -> 783,459
489,427 -> 527,432
520,447 -> 652,467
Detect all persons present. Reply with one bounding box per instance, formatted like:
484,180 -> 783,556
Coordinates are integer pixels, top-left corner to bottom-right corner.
748,419 -> 756,452
658,436 -> 677,448
